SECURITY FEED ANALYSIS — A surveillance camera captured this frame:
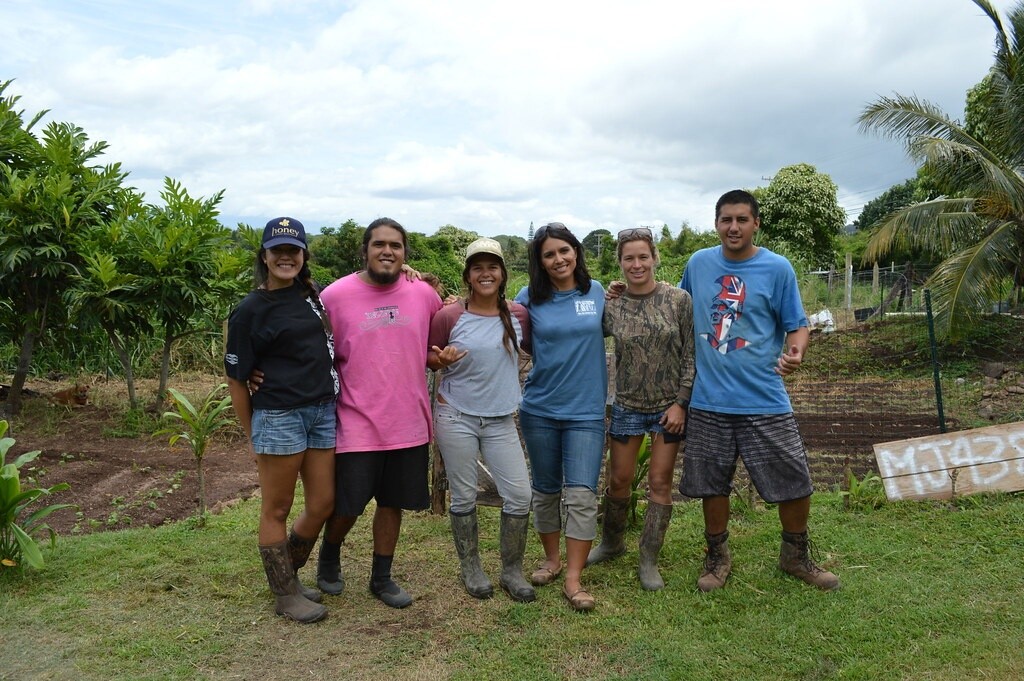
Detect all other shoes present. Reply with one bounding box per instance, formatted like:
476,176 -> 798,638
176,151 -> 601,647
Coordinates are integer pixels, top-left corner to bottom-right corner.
369,578 -> 413,608
532,564 -> 563,583
562,582 -> 595,610
317,542 -> 343,595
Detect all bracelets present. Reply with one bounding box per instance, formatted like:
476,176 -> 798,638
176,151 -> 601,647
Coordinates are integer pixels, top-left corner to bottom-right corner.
438,358 -> 447,366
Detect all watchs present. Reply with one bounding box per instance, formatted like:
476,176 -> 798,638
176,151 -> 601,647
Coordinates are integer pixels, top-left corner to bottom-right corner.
675,397 -> 688,410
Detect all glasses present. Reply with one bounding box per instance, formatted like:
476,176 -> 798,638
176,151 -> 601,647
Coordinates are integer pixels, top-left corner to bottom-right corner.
535,222 -> 565,239
618,228 -> 652,241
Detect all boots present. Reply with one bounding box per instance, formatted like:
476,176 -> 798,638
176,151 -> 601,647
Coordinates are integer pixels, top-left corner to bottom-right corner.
288,524 -> 324,603
499,508 -> 535,601
780,531 -> 839,590
583,486 -> 632,566
697,531 -> 731,590
637,497 -> 673,591
258,536 -> 328,623
449,506 -> 494,598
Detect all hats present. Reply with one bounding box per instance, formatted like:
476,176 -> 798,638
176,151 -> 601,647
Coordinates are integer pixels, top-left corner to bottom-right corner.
262,217 -> 306,250
465,238 -> 505,267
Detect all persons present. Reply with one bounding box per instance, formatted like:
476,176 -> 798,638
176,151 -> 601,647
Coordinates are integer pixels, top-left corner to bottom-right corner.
813,297 -> 835,335
223,190 -> 840,623
